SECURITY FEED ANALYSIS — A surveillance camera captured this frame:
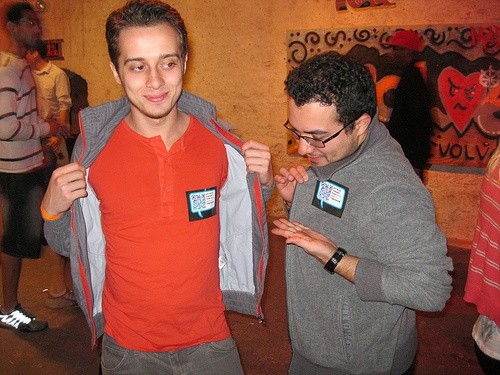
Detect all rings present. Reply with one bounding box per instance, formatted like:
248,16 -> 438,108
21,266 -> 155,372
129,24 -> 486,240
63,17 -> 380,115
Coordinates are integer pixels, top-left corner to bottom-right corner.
288,232 -> 293,238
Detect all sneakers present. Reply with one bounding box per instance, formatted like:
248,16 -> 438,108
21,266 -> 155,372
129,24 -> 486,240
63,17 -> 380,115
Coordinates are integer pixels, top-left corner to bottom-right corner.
43,288 -> 80,308
0,303 -> 48,332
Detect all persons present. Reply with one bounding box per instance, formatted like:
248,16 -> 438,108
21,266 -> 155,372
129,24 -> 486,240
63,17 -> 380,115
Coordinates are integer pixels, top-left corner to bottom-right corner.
39,0 -> 274,375
464,140 -> 500,375
1,3 -> 80,333
270,50 -> 453,375
25,38 -> 73,245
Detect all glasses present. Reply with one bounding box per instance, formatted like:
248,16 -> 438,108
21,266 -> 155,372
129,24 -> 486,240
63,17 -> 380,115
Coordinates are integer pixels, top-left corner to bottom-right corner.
13,19 -> 43,29
283,111 -> 370,148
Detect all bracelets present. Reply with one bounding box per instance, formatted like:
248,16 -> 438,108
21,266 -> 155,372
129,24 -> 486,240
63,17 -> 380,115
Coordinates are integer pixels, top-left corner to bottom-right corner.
40,205 -> 62,221
324,247 -> 347,275
